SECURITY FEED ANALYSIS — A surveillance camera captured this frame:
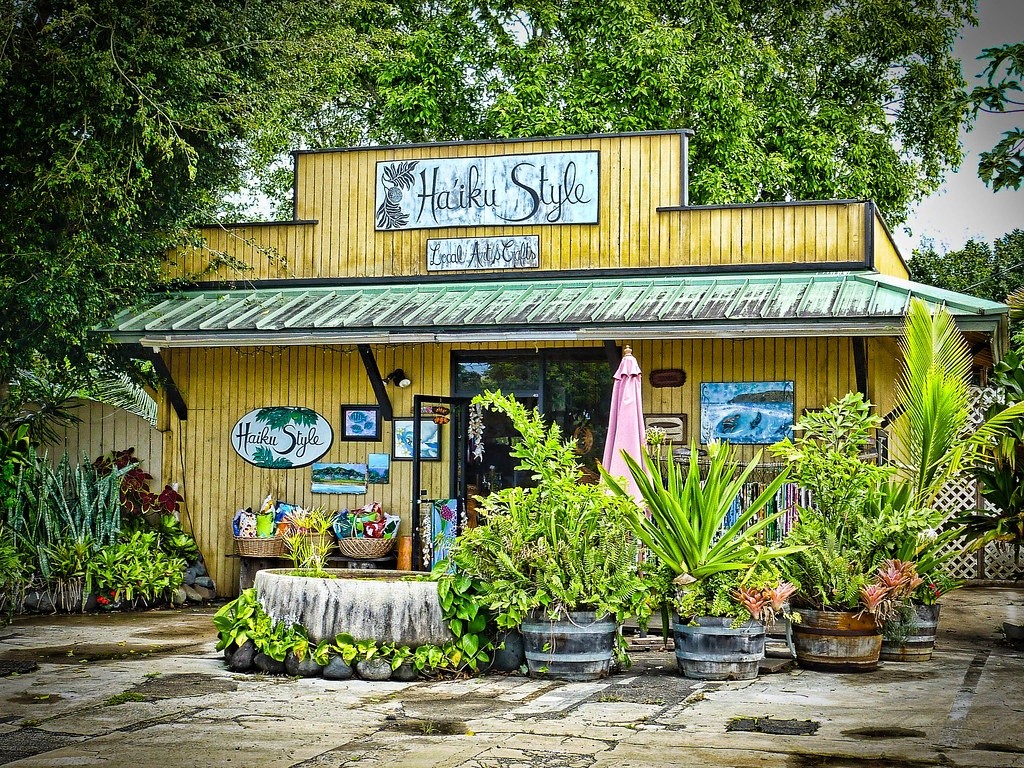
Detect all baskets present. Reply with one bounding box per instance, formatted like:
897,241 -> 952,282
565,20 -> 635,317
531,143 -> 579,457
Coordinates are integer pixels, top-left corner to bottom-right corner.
307,533 -> 335,556
233,535 -> 289,557
338,508 -> 398,558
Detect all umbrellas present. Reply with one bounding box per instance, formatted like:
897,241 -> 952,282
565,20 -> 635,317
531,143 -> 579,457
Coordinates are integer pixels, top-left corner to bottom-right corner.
599,344 -> 655,518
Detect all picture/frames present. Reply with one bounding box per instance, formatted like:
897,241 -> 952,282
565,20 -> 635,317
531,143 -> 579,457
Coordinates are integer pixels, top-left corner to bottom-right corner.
340,404 -> 382,443
391,416 -> 442,462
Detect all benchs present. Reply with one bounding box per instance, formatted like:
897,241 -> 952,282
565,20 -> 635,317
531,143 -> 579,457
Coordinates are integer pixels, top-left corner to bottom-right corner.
224,553 -> 392,595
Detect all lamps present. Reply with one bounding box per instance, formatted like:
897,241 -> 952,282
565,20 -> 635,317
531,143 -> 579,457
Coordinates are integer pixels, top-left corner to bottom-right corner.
382,369 -> 412,388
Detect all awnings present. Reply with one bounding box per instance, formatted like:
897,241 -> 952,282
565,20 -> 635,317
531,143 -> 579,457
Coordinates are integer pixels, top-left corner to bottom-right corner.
89,271 -> 1009,366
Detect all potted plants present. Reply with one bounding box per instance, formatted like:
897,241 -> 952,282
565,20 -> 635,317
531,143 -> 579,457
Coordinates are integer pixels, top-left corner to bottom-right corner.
594,437 -> 795,681
869,480 -> 960,663
452,484 -> 646,682
772,390 -> 918,671
